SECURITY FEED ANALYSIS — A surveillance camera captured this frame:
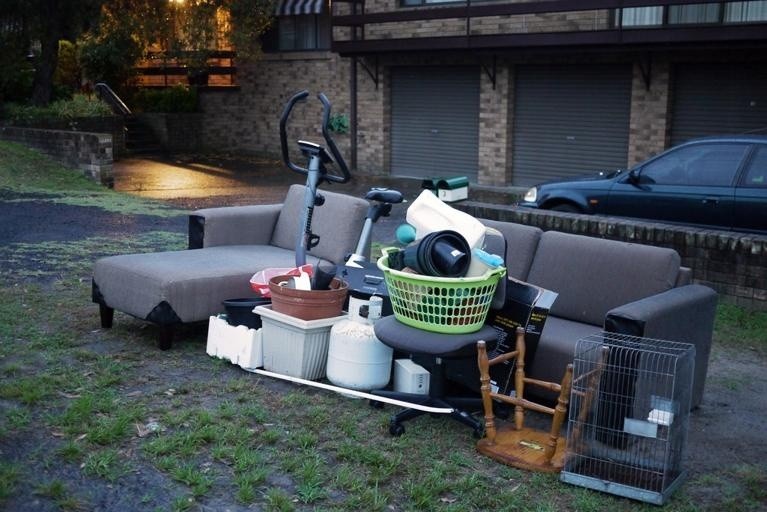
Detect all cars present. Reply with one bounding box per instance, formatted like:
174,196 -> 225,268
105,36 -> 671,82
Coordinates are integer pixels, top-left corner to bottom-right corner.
517,138 -> 767,233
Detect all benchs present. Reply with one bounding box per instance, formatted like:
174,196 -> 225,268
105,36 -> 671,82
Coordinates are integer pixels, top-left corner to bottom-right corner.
91,182 -> 374,372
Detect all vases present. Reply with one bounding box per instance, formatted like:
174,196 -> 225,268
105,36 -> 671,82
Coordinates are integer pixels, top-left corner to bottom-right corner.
249,302 -> 350,381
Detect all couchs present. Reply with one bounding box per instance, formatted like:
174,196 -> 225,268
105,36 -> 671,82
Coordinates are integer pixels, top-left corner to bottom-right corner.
362,214 -> 719,417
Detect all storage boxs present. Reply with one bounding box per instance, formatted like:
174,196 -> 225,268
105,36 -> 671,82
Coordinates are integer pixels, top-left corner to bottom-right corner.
411,275 -> 559,406
204,311 -> 266,370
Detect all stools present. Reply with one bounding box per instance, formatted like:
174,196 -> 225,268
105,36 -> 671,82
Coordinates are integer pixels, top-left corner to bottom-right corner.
473,325 -> 612,477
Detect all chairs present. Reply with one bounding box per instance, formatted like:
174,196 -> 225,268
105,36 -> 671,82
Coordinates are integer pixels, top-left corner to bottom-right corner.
367,225 -> 514,440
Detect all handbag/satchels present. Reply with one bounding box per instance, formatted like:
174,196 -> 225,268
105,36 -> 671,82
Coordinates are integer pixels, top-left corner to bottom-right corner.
249,264 -> 313,298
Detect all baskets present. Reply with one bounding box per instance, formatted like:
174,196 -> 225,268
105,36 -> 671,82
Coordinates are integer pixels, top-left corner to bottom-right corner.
376,246 -> 507,335
